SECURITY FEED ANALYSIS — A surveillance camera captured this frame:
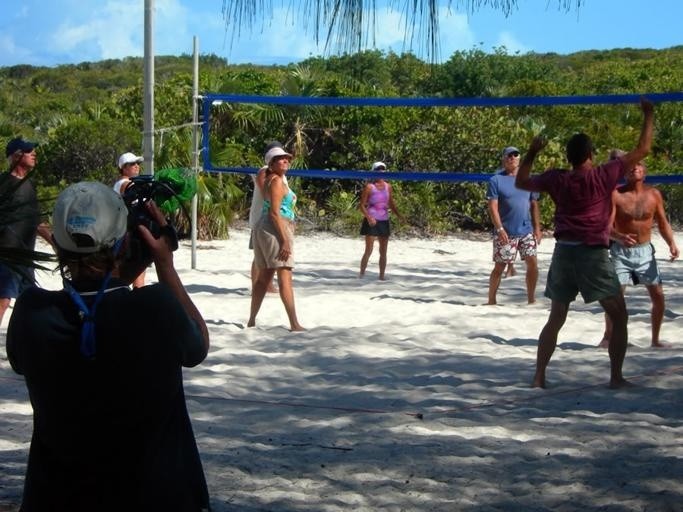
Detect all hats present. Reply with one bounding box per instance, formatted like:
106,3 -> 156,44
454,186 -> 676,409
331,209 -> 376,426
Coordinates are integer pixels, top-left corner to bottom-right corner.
609,149 -> 630,160
502,147 -> 520,159
371,162 -> 386,170
264,146 -> 293,165
117,152 -> 144,168
5,137 -> 40,155
53,181 -> 130,254
265,141 -> 285,152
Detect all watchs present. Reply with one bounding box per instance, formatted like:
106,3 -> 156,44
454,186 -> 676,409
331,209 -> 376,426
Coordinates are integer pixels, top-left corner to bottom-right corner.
496,227 -> 504,233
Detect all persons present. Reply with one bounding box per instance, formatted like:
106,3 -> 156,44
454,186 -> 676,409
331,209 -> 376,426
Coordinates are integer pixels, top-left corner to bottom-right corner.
504,246 -> 516,277
247,148 -> 307,332
0,139 -> 58,360
601,161 -> 678,350
488,146 -> 541,306
360,162 -> 399,280
514,97 -> 655,388
7,182 -> 211,512
250,143 -> 287,292
112,153 -> 150,292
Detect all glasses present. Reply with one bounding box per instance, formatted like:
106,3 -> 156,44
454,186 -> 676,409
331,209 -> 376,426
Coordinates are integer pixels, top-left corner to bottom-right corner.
505,152 -> 520,159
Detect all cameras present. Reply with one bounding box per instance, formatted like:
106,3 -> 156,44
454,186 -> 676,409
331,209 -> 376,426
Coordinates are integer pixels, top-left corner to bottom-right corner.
120,174 -> 185,286
